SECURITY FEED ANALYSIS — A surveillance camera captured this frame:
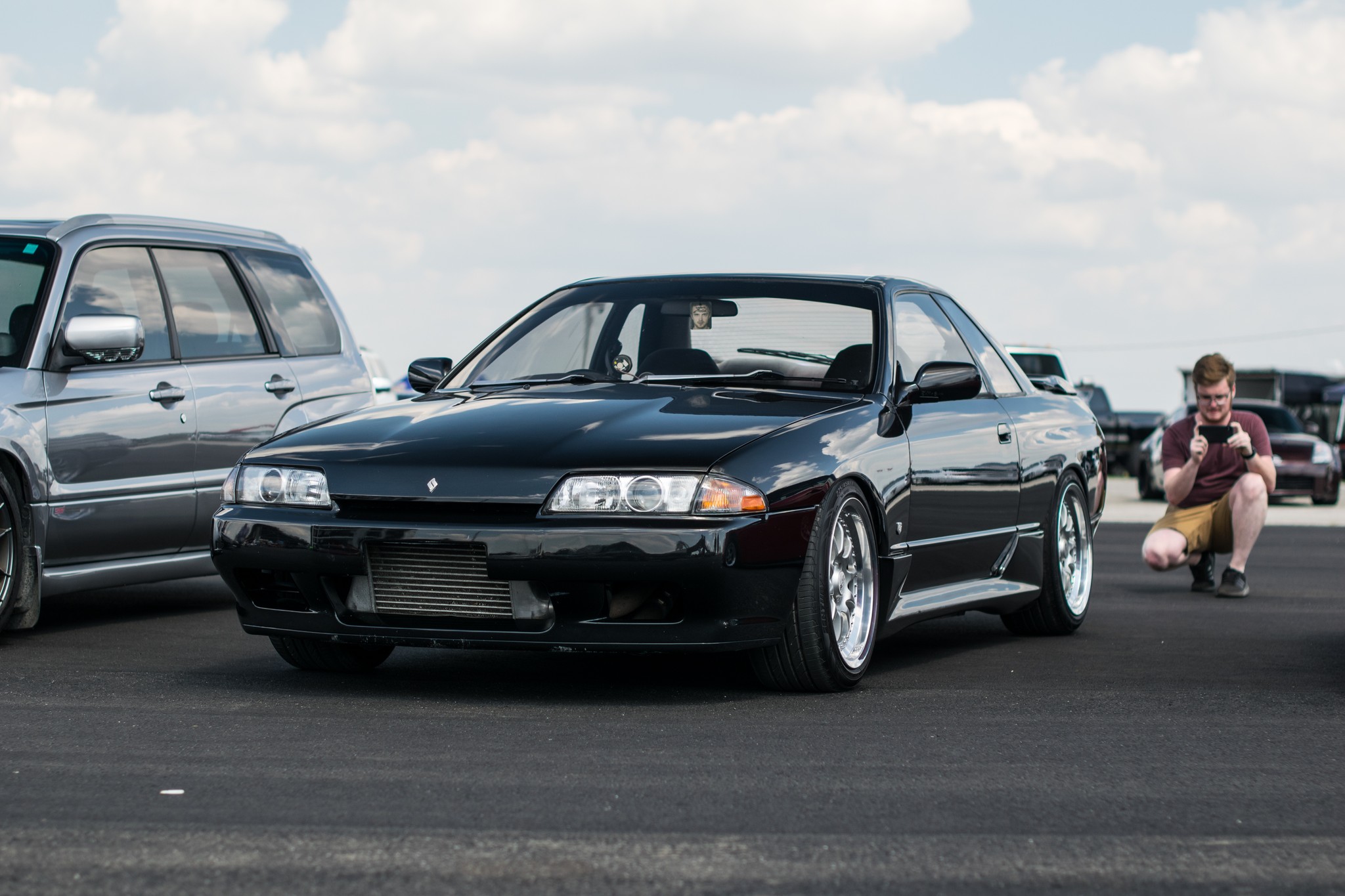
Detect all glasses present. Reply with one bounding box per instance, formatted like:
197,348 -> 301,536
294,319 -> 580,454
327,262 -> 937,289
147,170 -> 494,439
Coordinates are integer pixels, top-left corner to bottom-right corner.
1196,387 -> 1231,405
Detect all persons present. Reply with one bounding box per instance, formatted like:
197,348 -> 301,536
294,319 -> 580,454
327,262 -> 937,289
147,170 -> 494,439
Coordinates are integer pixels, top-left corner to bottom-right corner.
1141,352 -> 1276,598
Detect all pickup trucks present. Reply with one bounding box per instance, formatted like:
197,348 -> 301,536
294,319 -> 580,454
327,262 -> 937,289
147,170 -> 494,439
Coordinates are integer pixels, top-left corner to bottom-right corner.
1075,379 -> 1167,476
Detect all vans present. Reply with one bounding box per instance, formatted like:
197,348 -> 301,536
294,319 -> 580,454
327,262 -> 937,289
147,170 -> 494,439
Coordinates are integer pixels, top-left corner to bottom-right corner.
1177,369 -> 1345,450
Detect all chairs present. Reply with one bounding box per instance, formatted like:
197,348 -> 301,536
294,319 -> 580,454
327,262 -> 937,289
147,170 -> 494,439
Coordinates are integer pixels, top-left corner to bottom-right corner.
821,344 -> 904,393
632,348 -> 722,381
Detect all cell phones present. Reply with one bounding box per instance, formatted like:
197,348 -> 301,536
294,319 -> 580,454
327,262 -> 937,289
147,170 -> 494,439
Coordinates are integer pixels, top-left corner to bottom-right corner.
1197,425 -> 1234,444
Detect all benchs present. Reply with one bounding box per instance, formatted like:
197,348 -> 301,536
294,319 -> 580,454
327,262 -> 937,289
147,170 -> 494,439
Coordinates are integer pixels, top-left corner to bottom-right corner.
715,356 -> 831,388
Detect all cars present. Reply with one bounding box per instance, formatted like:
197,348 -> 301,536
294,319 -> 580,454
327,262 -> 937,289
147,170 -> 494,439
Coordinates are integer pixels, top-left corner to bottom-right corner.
362,347 -> 487,405
1001,341 -> 1071,386
1137,398 -> 1340,505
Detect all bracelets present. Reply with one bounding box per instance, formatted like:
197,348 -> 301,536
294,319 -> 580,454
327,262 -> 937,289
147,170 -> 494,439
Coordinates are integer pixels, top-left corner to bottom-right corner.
1240,446 -> 1257,459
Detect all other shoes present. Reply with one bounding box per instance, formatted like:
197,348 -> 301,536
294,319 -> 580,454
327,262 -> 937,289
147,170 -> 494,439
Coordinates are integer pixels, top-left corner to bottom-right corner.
1216,565 -> 1250,597
1188,549 -> 1217,592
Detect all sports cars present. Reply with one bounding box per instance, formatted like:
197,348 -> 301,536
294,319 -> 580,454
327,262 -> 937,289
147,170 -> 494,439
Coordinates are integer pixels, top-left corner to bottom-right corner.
211,271 -> 1112,691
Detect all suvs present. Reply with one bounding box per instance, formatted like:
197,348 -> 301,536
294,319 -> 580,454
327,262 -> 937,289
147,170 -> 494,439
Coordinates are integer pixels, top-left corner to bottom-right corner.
0,214 -> 373,639
